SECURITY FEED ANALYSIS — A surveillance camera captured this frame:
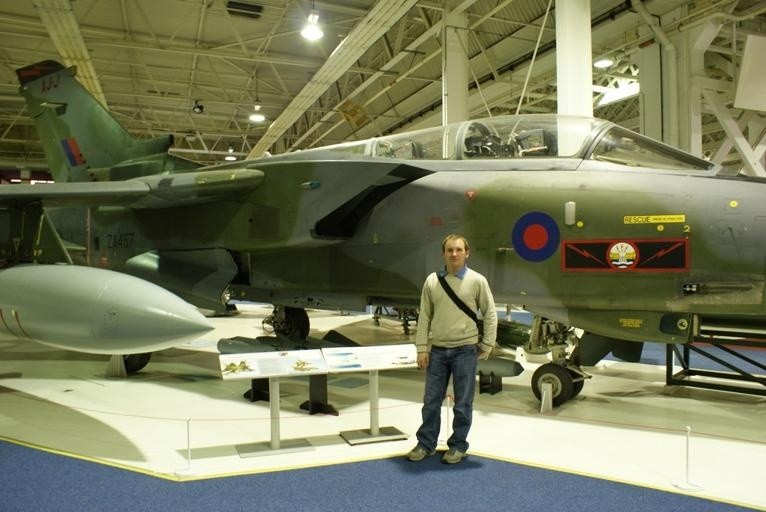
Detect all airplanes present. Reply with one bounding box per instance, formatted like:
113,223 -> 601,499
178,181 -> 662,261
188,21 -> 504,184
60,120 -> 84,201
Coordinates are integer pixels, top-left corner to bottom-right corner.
3,59 -> 766,403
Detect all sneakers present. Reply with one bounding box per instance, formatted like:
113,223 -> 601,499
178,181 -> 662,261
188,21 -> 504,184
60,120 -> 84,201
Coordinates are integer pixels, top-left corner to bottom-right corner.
406,444 -> 436,461
440,448 -> 466,464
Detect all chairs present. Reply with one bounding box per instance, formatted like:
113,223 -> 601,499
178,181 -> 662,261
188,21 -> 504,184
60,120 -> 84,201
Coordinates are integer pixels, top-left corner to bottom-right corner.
412,142 -> 425,159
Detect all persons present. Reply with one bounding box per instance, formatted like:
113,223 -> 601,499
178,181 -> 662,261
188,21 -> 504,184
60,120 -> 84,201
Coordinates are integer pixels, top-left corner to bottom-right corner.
402,232 -> 499,465
